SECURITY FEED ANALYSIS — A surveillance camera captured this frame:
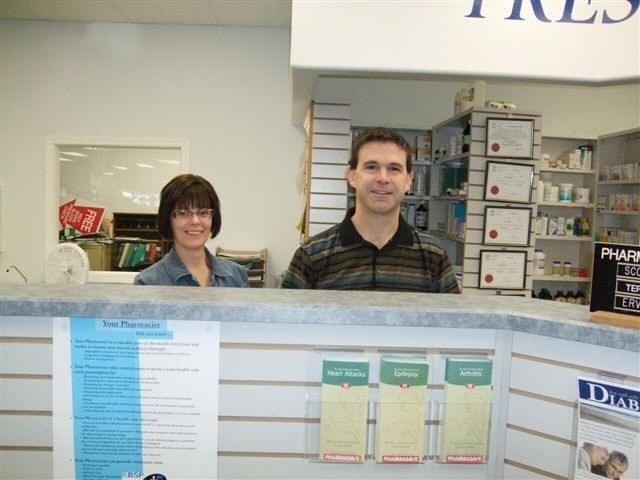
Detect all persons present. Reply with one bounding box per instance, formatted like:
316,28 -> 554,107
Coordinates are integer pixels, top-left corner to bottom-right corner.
576,440 -> 609,476
280,126 -> 461,293
133,174 -> 253,287
586,451 -> 631,480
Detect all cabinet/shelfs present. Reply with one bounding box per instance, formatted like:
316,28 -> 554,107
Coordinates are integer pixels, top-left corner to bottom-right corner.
111,211 -> 164,272
348,106 -> 640,307
215,246 -> 268,284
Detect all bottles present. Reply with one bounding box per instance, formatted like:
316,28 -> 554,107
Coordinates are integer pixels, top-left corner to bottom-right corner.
563,261 -> 573,277
534,249 -> 546,276
457,122 -> 470,154
552,261 -> 561,277
536,212 -> 542,234
415,202 -> 427,230
532,289 -> 585,305
558,183 -> 590,204
414,167 -> 425,196
538,179 -> 544,201
580,217 -> 590,238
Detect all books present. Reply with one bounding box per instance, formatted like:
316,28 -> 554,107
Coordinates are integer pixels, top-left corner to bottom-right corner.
117,242 -> 157,269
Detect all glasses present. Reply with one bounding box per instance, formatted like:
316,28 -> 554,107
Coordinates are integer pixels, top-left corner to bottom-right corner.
170,208 -> 215,221
